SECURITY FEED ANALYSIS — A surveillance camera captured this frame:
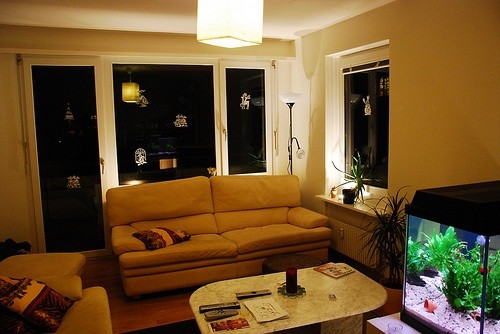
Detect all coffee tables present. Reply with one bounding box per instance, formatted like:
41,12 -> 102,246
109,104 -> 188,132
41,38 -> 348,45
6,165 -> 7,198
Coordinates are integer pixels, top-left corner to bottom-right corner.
189,263 -> 388,334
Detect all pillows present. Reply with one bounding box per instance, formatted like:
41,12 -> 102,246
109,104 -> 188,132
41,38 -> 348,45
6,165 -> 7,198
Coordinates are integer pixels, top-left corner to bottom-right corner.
133,228 -> 188,251
0,278 -> 72,334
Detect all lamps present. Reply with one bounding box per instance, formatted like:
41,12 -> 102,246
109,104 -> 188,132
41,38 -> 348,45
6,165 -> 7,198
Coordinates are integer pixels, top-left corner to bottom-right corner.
279,94 -> 305,174
121,68 -> 140,102
197,0 -> 263,47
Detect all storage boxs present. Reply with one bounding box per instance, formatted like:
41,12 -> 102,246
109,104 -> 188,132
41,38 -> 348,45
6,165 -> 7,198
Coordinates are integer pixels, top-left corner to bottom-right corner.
400,181 -> 500,334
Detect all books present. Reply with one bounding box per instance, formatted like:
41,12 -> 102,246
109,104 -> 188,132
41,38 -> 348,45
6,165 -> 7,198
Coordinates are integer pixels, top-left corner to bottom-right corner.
313,262 -> 355,279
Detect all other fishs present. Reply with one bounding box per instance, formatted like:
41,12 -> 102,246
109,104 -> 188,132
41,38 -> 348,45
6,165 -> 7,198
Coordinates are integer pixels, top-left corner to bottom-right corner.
468,310 -> 480,321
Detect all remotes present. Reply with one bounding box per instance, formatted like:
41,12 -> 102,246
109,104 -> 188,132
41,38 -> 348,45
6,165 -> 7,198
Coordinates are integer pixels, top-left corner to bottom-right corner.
199,302 -> 240,313
205,310 -> 238,321
236,290 -> 271,298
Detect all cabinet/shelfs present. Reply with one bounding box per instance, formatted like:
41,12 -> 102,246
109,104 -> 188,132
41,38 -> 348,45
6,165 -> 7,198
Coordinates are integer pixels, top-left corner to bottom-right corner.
314,194 -> 387,270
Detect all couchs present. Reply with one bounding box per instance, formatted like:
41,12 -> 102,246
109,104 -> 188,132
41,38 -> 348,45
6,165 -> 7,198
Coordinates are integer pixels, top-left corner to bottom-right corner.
106,175 -> 331,301
0,253 -> 114,334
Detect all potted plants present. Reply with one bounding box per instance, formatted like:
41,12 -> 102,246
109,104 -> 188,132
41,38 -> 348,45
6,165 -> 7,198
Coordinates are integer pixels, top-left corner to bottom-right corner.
353,185 -> 412,314
329,149 -> 384,204
